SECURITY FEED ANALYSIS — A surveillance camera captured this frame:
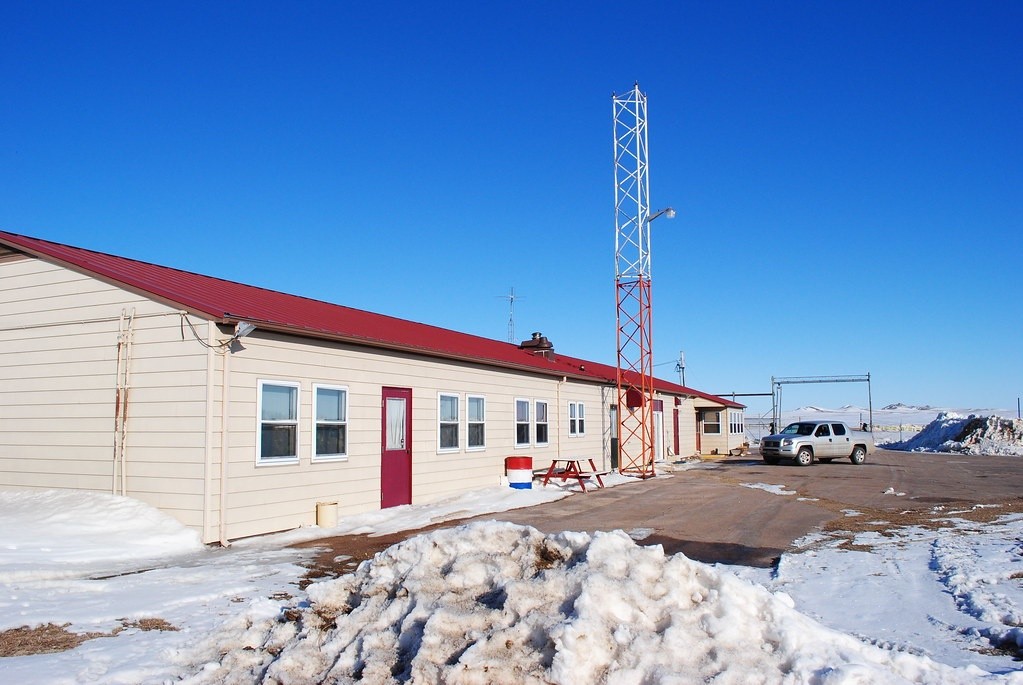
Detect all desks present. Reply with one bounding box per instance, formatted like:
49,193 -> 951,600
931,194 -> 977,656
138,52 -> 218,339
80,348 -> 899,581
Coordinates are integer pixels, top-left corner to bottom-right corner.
532,458 -> 609,493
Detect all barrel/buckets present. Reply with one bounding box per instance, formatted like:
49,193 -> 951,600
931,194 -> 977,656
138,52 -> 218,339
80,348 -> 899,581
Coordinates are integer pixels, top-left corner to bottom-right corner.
506,456 -> 532,489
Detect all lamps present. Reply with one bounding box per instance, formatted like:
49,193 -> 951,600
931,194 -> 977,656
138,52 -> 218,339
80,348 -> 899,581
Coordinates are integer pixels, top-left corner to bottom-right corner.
230,321 -> 257,343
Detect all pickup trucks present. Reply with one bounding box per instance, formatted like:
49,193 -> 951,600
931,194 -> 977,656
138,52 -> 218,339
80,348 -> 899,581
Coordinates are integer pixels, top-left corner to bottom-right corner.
760,422 -> 872,469
